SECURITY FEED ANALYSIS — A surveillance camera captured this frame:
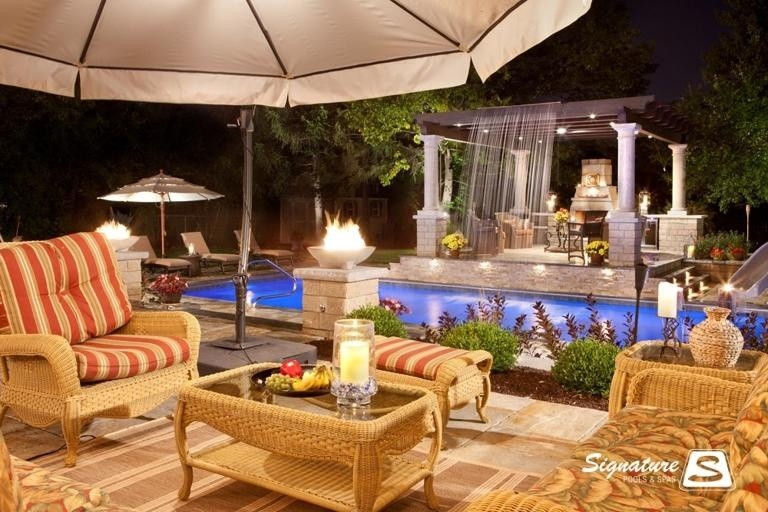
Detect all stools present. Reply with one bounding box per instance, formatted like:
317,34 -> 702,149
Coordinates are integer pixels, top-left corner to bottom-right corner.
373,335 -> 494,451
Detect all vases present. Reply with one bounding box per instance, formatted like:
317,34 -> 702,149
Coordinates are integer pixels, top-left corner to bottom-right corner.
591,252 -> 604,266
732,253 -> 745,260
448,249 -> 461,259
160,292 -> 181,304
712,256 -> 722,260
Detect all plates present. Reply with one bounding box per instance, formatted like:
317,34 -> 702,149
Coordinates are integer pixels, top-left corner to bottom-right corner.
252,365 -> 333,397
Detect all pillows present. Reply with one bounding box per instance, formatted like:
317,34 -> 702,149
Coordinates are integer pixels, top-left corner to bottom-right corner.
0,231 -> 134,345
0,428 -> 18,512
727,361 -> 768,481
718,436 -> 768,512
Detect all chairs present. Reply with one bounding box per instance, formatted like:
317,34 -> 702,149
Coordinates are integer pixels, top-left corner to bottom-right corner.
127,228 -> 295,277
0,309 -> 203,469
567,210 -> 608,266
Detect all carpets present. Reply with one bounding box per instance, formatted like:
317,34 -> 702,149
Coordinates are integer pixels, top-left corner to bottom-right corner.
26,410 -> 546,512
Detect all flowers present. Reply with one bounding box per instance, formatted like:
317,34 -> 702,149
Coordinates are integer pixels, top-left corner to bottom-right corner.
148,274 -> 189,295
585,240 -> 609,255
730,247 -> 746,254
441,234 -> 464,251
709,248 -> 725,257
555,207 -> 569,222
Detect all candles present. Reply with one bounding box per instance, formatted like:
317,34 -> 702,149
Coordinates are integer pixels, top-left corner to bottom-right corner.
339,339 -> 370,384
657,281 -> 678,319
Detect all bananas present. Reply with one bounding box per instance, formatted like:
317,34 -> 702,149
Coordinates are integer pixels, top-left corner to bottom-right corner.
292,364 -> 333,394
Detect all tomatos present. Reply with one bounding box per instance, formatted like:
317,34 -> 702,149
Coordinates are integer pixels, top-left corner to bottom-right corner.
281,359 -> 301,377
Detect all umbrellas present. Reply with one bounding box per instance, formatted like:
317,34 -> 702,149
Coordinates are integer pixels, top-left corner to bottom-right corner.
96,172 -> 226,258
0,0 -> 591,114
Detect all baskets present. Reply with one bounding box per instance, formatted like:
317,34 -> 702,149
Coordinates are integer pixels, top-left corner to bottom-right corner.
157,292 -> 182,304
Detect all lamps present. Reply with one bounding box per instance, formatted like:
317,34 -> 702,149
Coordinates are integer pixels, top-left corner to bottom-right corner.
307,209 -> 376,269
94,218 -> 141,252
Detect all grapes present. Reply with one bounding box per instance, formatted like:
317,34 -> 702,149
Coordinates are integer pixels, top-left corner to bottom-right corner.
265,374 -> 292,393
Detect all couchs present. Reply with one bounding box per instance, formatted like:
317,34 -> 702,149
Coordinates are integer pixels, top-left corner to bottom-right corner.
11,454 -> 132,512
460,369 -> 768,512
